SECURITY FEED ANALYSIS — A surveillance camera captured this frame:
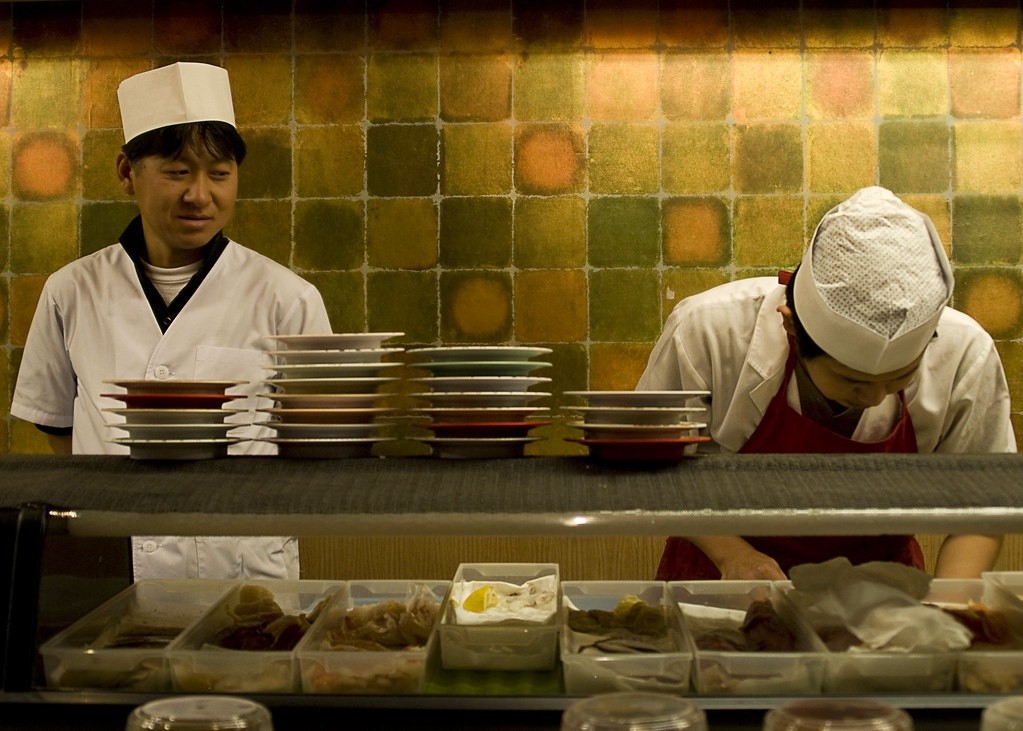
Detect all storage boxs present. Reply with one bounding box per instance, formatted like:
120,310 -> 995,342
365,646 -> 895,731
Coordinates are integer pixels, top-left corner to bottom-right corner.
40,562 -> 1023,698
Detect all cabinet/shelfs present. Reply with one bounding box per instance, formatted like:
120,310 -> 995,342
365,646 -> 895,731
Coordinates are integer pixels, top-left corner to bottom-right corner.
0,454 -> 1023,731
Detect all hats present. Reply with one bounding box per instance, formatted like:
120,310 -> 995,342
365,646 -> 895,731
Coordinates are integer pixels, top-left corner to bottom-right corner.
793,186 -> 956,376
117,62 -> 237,146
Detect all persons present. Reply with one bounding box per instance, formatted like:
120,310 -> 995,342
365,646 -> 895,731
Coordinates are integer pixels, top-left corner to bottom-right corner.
635,187 -> 1017,580
10,61 -> 332,611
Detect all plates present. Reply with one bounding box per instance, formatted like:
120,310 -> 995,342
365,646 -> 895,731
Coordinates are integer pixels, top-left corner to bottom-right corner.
406,344 -> 555,459
97,375 -> 255,461
558,390 -> 712,466
253,332 -> 405,459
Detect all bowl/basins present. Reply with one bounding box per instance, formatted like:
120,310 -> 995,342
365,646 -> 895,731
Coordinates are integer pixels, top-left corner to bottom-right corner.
39,560 -> 1023,731
